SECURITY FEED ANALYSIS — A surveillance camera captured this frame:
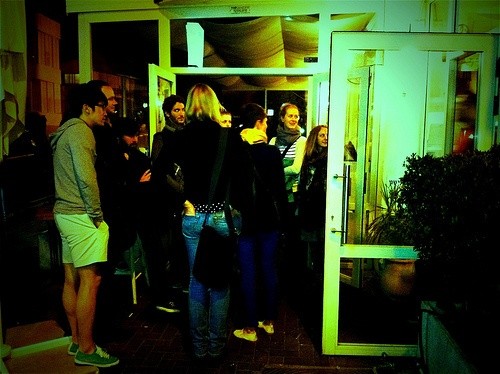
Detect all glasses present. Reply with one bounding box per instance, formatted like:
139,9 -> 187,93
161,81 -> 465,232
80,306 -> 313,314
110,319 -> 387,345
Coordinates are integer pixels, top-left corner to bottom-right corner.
92,103 -> 107,109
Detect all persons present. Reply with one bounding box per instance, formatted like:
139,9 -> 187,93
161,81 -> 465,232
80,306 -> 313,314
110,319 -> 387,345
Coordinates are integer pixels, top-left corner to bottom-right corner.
87,80 -> 357,358
48,87 -> 120,368
453,90 -> 478,154
8,112 -> 56,212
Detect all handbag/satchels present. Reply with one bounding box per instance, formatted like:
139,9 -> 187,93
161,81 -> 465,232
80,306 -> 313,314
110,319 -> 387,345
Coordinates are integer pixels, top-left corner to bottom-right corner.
191,226 -> 241,290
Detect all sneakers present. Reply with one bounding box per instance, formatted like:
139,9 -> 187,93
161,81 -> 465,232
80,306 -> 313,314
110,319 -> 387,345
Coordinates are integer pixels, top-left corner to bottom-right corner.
74,345 -> 120,368
233,328 -> 258,342
208,357 -> 222,366
193,359 -> 207,368
67,342 -> 79,356
257,319 -> 275,334
156,299 -> 182,313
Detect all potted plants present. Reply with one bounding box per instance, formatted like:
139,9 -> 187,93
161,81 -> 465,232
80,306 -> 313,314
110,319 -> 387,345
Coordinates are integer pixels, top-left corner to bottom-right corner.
364,179 -> 415,300
